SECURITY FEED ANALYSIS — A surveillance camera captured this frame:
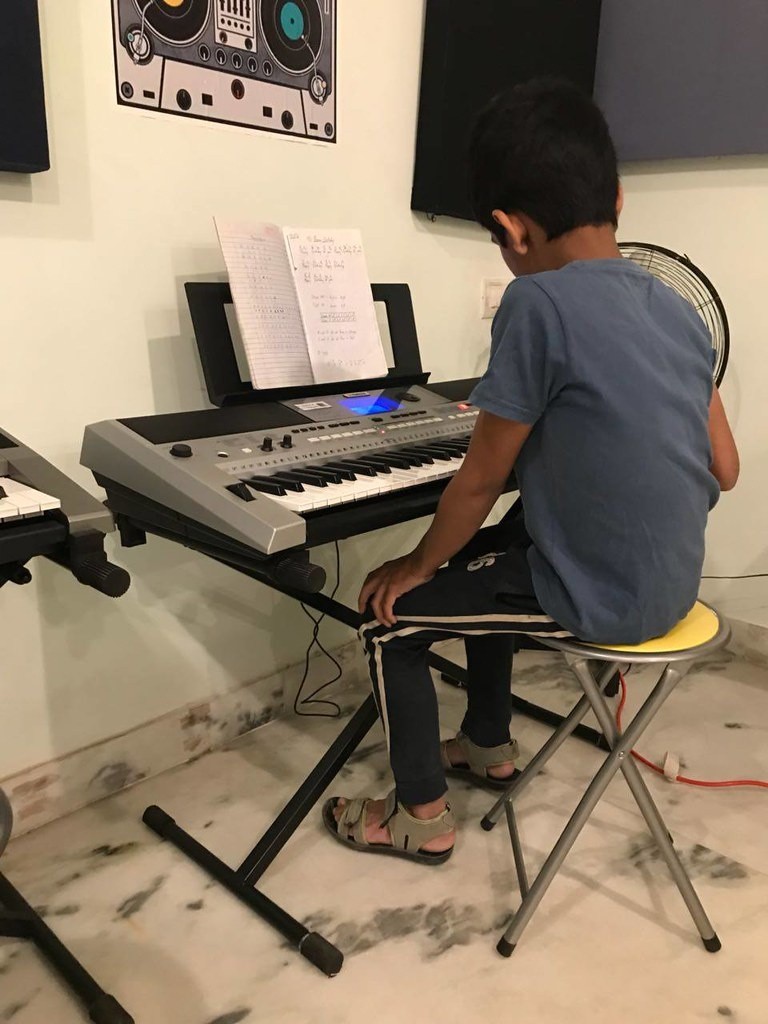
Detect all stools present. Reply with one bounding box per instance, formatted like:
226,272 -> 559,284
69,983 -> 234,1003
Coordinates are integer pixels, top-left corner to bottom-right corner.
479,598 -> 731,958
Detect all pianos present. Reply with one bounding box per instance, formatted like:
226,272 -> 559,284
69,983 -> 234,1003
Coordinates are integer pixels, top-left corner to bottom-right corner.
0,425 -> 133,598
78,375 -> 524,596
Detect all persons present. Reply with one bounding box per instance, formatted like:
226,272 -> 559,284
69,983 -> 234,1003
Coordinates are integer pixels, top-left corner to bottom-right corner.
325,79 -> 739,865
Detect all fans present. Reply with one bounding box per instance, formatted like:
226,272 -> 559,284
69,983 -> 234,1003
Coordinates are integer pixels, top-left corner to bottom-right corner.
617,241 -> 730,389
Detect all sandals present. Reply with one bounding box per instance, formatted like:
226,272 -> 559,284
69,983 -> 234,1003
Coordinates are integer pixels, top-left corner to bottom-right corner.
438,730 -> 524,791
322,789 -> 458,865
